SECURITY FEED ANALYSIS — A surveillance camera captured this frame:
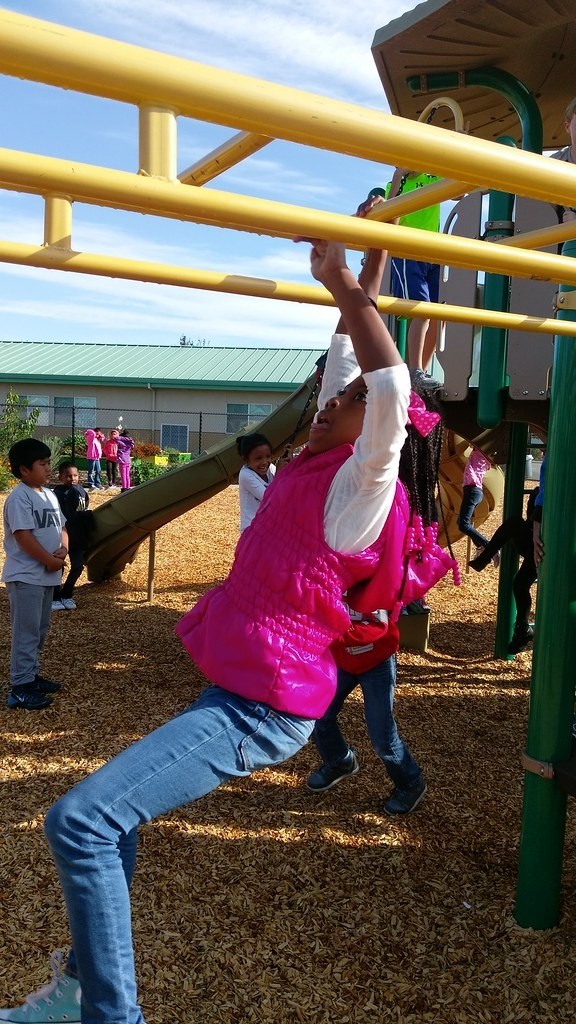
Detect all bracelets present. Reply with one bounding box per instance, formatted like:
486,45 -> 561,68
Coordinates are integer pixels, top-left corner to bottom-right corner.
367,296 -> 377,310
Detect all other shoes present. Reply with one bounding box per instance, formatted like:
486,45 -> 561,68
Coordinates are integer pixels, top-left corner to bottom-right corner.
467,557 -> 491,573
492,550 -> 500,569
507,626 -> 534,654
472,547 -> 485,559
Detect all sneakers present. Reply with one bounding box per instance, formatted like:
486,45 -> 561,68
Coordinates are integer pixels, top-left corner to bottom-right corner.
0,950 -> 82,1024
30,674 -> 60,693
383,777 -> 428,815
7,688 -> 53,710
307,749 -> 359,791
411,366 -> 442,389
60,598 -> 76,609
51,601 -> 65,610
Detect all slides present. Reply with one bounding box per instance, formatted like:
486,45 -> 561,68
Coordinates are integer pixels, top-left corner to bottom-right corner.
74,364 -> 325,583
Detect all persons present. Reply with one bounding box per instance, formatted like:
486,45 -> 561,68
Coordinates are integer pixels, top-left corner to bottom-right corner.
102,429 -> 120,488
468,449 -> 547,654
305,583 -> 426,815
1,439 -> 71,709
385,121 -> 470,389
456,446 -> 500,567
114,429 -> 135,493
50,461 -> 90,610
0,195 -> 445,1024
84,428 -> 105,489
547,95 -> 576,255
235,433 -> 295,534
